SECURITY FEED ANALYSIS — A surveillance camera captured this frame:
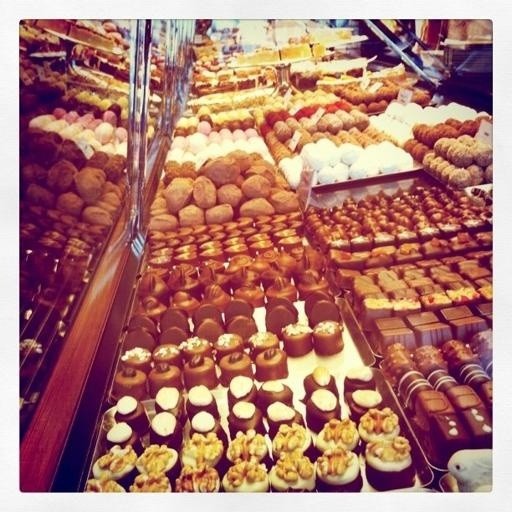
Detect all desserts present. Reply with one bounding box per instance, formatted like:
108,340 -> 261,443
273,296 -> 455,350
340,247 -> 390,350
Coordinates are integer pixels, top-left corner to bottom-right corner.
86,27 -> 493,493
19,19 -> 166,415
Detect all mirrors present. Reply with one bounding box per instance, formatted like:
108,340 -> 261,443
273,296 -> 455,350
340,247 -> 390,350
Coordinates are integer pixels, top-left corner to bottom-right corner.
20,18 -> 198,444
